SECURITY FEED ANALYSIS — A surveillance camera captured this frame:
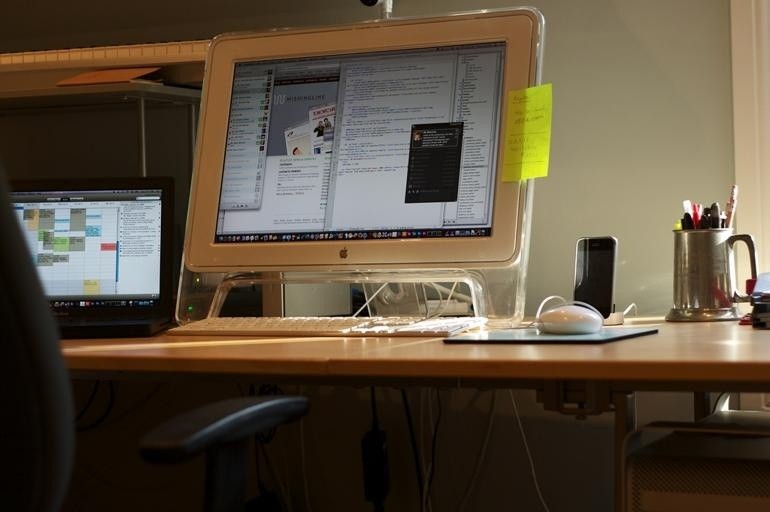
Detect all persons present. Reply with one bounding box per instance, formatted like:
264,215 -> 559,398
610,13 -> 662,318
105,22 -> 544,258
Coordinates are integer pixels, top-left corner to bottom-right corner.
314,121 -> 325,137
413,132 -> 422,146
323,118 -> 332,128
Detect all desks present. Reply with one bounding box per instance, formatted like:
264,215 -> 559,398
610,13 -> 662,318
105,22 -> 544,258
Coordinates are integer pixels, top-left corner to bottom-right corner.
56,314 -> 770,512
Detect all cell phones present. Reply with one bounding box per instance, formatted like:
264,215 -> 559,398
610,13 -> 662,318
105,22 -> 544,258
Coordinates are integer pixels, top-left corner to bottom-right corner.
574,235 -> 617,320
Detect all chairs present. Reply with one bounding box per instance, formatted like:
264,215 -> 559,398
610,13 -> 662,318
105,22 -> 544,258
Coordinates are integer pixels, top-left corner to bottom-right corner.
0,175 -> 311,512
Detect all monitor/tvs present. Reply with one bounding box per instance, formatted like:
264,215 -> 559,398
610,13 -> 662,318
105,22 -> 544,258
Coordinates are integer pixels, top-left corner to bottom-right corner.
182,5 -> 547,328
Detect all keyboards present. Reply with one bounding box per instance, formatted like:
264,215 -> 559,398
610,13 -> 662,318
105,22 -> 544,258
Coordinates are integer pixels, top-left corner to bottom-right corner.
164,315 -> 493,342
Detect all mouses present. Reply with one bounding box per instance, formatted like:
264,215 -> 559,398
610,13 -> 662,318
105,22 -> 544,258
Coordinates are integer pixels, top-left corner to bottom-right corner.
536,304 -> 604,334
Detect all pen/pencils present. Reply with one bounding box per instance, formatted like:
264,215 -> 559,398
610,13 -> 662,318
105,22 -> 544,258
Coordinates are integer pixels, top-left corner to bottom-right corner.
675,184 -> 737,229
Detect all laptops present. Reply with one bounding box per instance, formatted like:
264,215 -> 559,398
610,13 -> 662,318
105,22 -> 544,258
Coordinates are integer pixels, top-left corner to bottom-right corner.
3,175 -> 178,340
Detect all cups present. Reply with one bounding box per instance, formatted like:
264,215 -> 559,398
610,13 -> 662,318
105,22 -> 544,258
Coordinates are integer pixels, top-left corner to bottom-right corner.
666,228 -> 758,321
